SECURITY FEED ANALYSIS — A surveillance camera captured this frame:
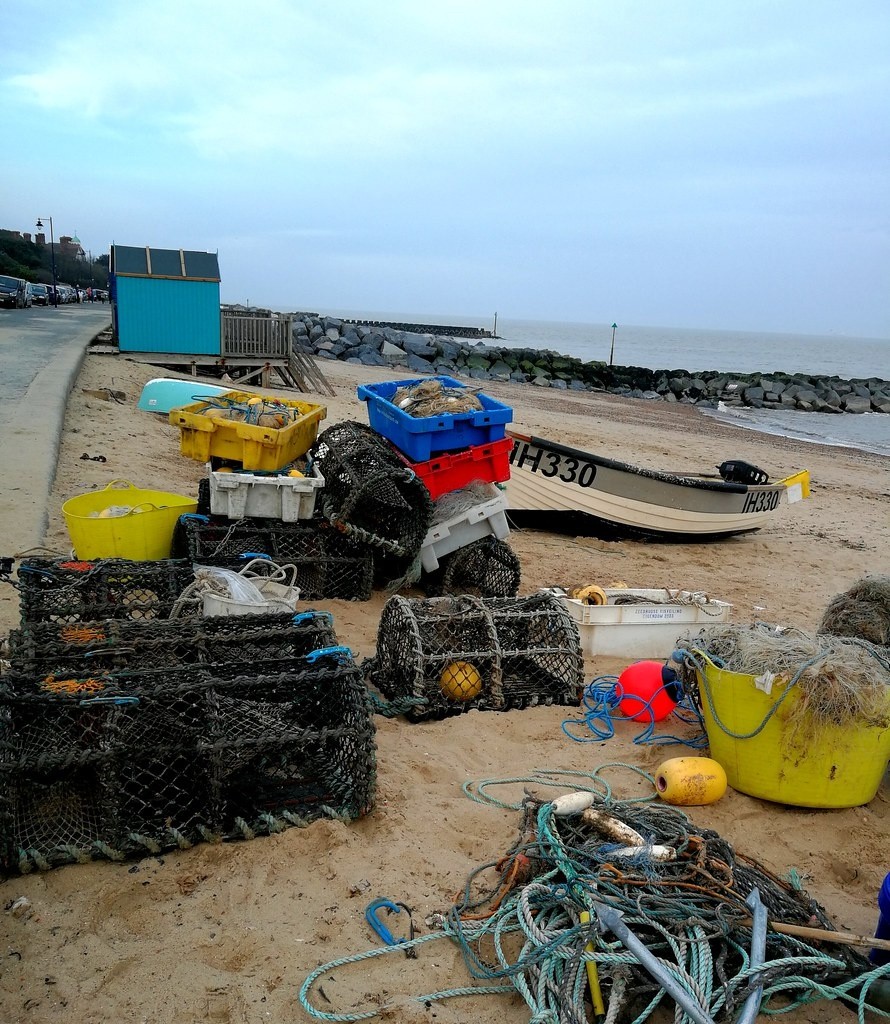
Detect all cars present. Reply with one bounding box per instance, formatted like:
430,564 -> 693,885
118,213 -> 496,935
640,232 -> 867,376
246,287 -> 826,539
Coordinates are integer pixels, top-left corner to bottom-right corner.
0,274 -> 110,309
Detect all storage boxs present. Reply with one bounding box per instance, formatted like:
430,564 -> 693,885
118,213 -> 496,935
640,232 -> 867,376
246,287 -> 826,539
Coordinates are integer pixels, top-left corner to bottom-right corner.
205,452 -> 325,523
358,376 -> 513,463
539,587 -> 733,658
385,437 -> 512,505
420,481 -> 511,573
170,390 -> 329,472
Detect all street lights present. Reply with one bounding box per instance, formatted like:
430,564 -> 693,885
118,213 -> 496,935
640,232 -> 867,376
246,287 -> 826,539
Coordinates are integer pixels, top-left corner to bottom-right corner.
35,216 -> 58,308
609,323 -> 619,365
82,249 -> 93,303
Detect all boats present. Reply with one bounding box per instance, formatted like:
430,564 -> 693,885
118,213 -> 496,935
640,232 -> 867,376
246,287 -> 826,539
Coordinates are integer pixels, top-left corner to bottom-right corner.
136,376 -> 240,414
504,428 -> 811,544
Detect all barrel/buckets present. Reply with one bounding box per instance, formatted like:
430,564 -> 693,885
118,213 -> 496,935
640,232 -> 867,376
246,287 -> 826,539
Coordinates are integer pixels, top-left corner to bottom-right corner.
693,647 -> 890,806
62,480 -> 198,581
204,579 -> 298,618
